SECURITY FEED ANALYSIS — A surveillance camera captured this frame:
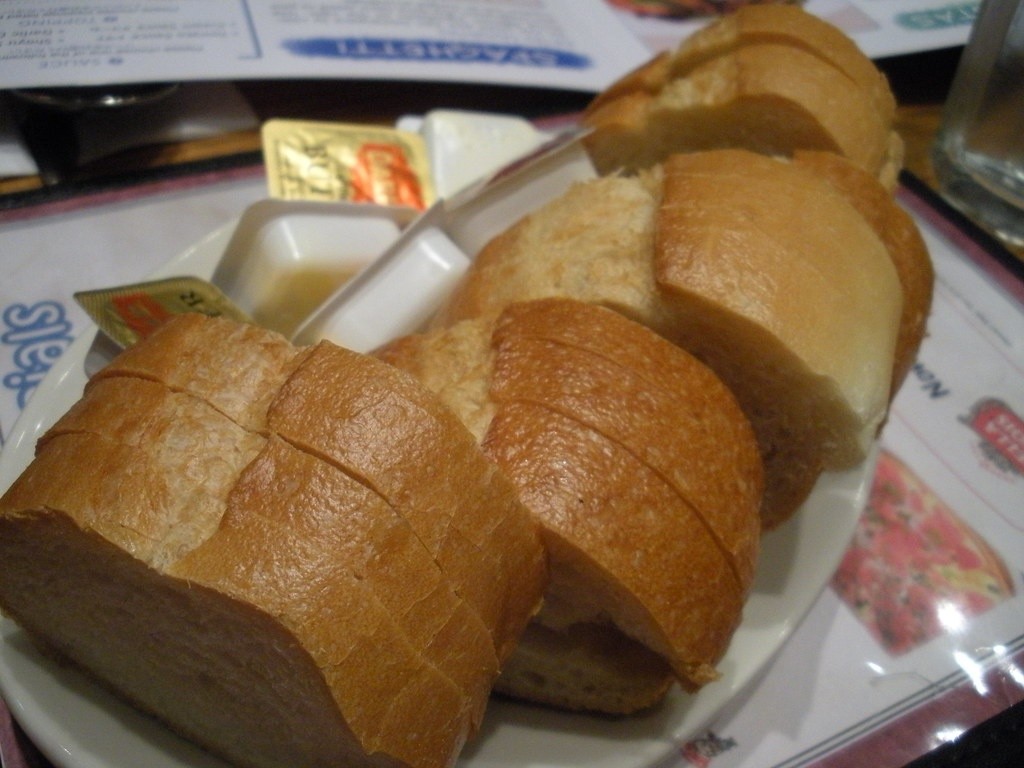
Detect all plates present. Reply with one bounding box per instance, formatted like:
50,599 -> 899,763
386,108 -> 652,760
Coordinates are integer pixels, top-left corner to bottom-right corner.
0,196 -> 859,768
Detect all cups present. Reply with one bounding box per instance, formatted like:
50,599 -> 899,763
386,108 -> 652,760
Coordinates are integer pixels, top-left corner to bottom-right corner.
928,0 -> 1024,246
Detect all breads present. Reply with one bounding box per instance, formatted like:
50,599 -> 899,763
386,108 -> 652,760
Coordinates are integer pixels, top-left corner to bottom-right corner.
1,3 -> 937,768
